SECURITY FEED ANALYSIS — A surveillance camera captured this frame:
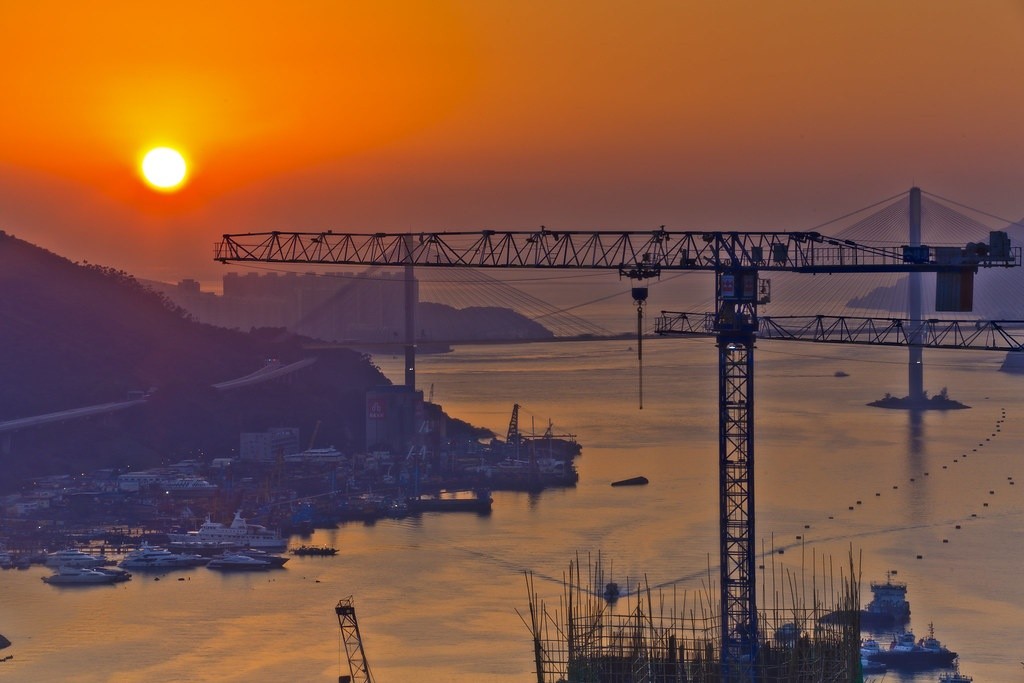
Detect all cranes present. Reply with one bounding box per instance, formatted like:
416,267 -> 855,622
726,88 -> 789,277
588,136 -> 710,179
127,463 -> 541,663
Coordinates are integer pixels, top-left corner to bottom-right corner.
654,309 -> 1024,353
211,224 -> 1024,683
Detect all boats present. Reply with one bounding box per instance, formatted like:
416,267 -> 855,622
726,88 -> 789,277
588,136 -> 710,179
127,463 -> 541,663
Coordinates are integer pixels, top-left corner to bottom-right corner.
773,621 -> 801,641
293,544 -> 340,555
119,539 -> 213,568
237,547 -> 290,569
165,507 -> 289,556
40,565 -> 133,585
860,622 -> 959,674
206,554 -> 272,572
44,547 -> 107,568
863,569 -> 911,625
